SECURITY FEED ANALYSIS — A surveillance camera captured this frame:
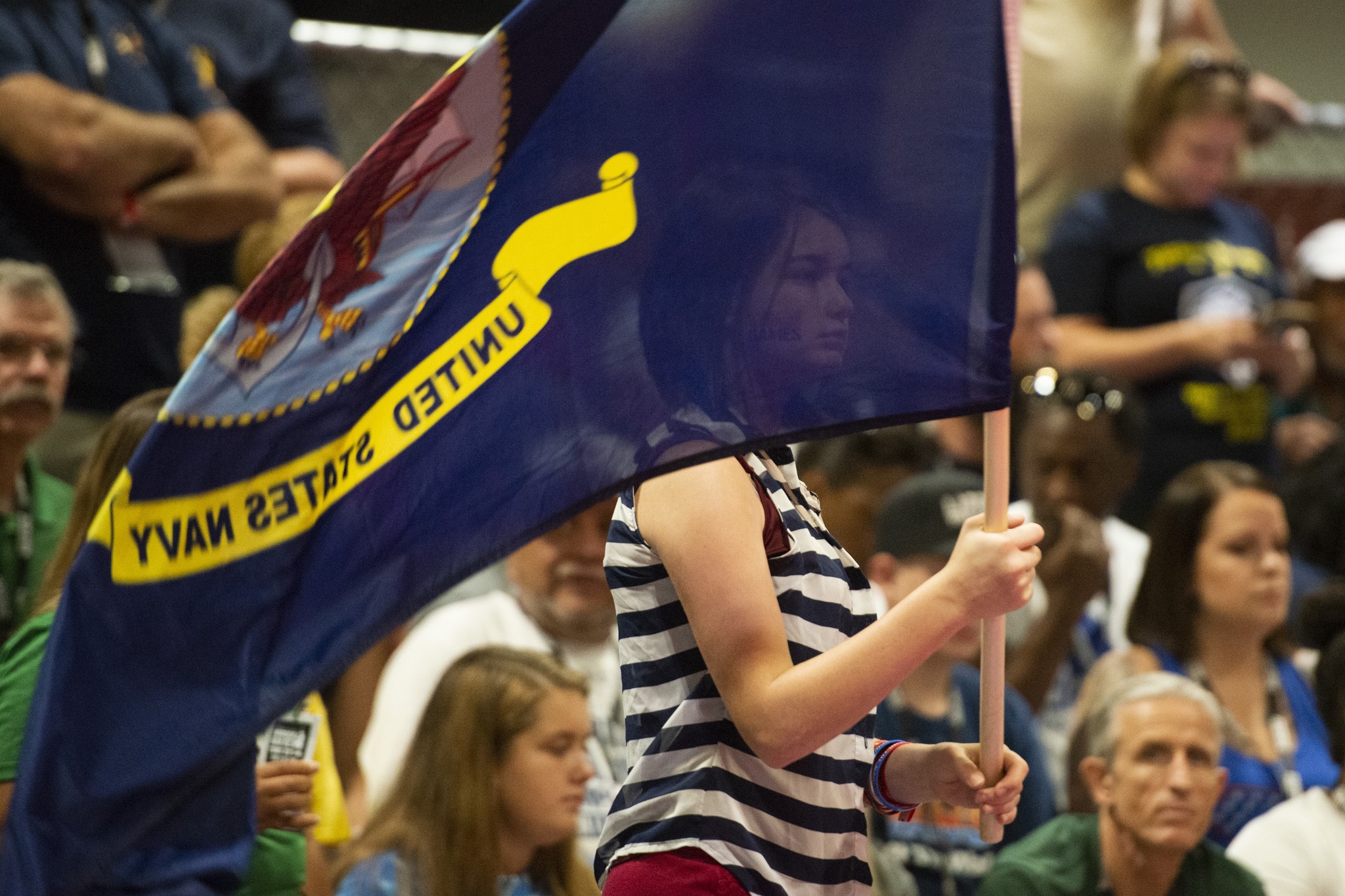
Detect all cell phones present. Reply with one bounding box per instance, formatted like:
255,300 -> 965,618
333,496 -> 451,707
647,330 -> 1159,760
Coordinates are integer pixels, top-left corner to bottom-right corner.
257,709 -> 319,766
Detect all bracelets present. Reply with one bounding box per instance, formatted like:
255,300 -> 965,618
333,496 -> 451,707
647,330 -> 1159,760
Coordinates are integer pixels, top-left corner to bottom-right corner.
872,741 -> 923,812
868,739 -> 916,816
863,740 -> 917,822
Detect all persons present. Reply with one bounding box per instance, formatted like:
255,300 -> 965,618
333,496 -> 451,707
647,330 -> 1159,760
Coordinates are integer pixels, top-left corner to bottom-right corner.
0,0 -> 1345,896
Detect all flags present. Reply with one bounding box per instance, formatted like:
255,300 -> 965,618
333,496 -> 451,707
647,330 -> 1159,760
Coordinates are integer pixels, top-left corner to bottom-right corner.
0,0 -> 1020,896
